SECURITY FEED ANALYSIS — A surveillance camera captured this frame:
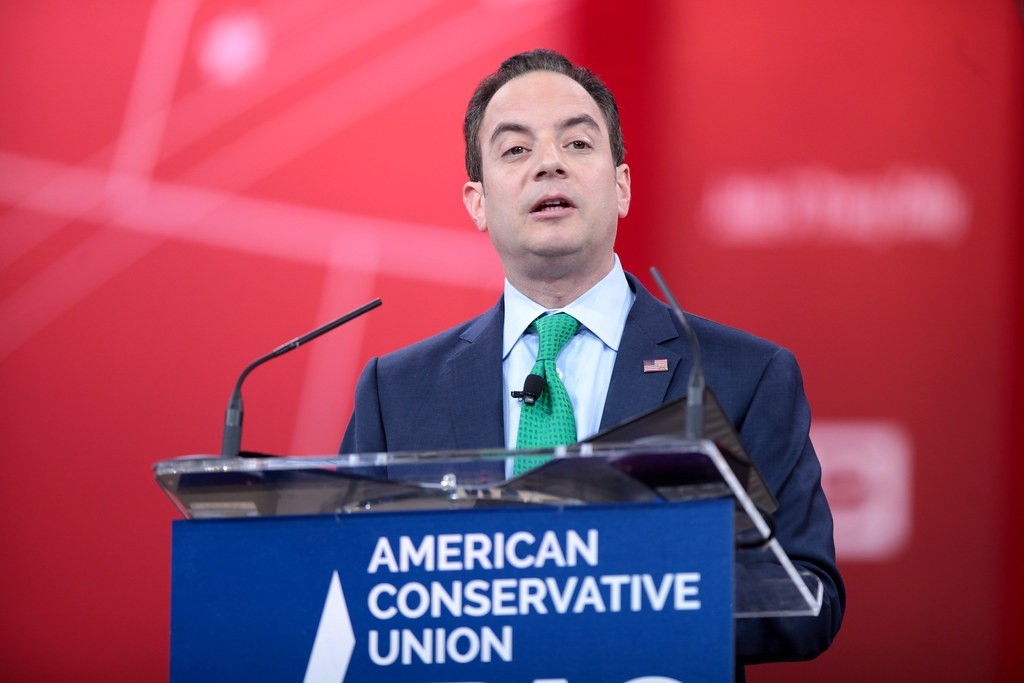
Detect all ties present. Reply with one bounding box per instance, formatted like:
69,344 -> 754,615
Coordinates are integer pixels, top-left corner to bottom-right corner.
512,313 -> 581,477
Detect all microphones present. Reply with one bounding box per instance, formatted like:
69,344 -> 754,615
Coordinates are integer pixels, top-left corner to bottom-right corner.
650,267 -> 706,440
522,374 -> 544,406
222,299 -> 382,457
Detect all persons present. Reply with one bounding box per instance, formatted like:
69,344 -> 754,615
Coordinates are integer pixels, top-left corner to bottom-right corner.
334,48 -> 846,683
